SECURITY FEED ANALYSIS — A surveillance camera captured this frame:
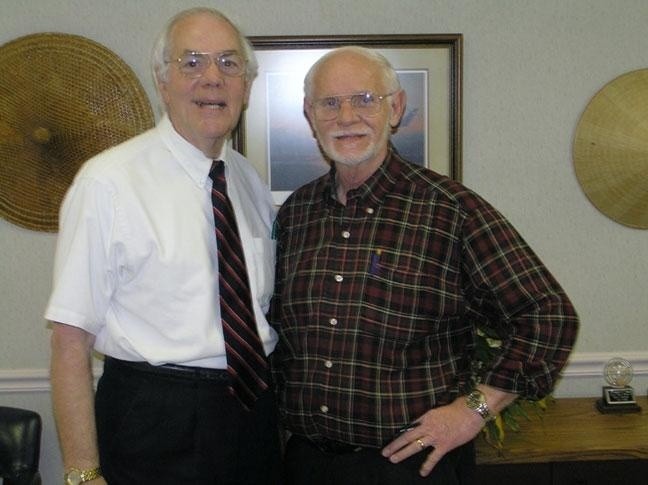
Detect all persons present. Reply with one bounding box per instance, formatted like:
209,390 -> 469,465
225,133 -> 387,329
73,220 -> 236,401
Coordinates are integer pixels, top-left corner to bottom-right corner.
44,7 -> 284,485
270,45 -> 583,484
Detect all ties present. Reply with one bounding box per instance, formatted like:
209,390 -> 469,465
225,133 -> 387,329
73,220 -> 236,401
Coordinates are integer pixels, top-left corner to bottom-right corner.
209,156 -> 271,411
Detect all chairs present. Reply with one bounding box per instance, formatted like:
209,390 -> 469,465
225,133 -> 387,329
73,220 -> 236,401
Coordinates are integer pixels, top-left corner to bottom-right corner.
0,405 -> 42,485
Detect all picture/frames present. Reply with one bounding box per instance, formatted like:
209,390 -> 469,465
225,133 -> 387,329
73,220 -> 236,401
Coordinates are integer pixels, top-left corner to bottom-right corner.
230,33 -> 464,211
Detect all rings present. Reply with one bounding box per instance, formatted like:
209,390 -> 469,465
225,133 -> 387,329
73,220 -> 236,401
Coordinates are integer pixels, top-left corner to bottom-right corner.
416,440 -> 426,449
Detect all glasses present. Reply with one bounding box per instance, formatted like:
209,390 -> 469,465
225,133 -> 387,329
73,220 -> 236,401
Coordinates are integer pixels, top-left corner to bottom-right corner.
164,49 -> 244,76
307,93 -> 396,116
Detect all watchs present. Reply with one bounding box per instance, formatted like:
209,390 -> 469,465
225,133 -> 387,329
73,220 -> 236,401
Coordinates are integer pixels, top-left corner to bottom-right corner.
62,466 -> 102,485
464,390 -> 493,421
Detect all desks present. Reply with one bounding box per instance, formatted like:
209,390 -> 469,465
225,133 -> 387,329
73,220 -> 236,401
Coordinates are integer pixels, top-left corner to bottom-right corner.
473,395 -> 648,485
